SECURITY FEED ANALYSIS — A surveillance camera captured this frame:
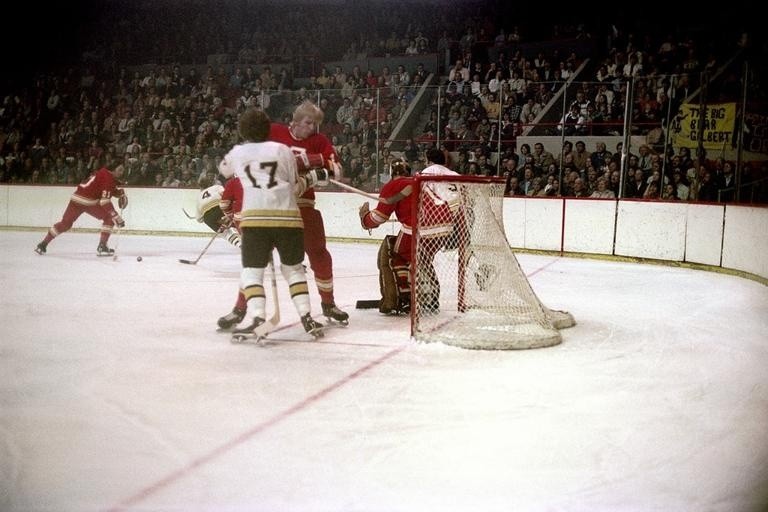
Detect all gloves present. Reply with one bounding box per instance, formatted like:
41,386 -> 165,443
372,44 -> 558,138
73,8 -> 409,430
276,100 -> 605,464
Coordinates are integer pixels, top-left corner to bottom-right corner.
118,189 -> 128,209
110,211 -> 125,227
358,202 -> 370,230
306,168 -> 329,187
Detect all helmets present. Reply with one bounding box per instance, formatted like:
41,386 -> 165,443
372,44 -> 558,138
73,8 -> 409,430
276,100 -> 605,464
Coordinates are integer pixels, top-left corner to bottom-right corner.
390,157 -> 411,180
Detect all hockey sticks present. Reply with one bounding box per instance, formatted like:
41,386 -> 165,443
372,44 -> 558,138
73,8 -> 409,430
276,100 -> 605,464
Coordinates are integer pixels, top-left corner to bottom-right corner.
112,203 -> 124,262
177,219 -> 229,264
182,207 -> 200,219
253,250 -> 280,336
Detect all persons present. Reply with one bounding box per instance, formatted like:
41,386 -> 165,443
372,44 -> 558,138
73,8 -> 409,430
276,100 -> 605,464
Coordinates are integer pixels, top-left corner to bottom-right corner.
359,157 -> 455,317
216,101 -> 328,340
32,160 -> 128,254
192,170 -> 240,247
2,1 -> 768,203
419,149 -> 494,291
217,99 -> 351,329
219,173 -> 244,234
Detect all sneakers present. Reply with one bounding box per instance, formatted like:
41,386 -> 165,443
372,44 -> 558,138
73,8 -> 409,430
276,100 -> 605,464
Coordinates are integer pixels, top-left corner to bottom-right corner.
97,242 -> 114,252
398,295 -> 411,312
37,241 -> 48,252
217,303 -> 348,335
